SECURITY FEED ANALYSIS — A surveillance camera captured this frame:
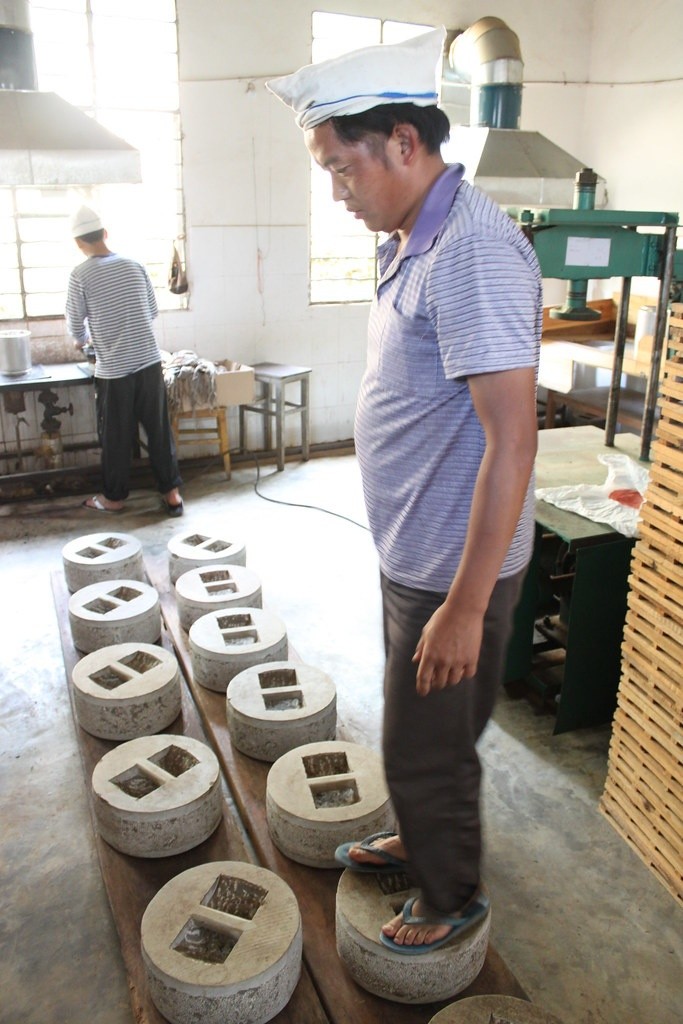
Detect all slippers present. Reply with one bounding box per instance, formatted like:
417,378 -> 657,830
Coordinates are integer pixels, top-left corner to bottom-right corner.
334,831 -> 413,873
81,493 -> 126,515
163,492 -> 184,516
379,891 -> 489,953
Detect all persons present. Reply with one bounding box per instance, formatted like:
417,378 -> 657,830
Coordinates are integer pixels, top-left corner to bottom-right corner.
62,202 -> 184,520
259,26 -> 544,956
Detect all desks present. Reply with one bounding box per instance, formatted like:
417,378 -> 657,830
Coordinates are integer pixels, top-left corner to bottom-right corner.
1,360 -> 156,485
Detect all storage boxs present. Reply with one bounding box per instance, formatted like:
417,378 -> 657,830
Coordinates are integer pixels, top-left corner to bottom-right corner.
178,358 -> 255,414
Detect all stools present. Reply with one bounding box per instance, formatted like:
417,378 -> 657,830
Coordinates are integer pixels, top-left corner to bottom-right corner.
240,361 -> 313,472
169,406 -> 231,481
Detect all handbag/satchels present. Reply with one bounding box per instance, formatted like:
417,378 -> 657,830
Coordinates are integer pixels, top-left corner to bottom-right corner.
169,240 -> 188,294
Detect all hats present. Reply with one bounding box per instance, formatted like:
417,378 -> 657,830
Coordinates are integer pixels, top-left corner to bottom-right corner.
266,26 -> 449,132
71,205 -> 104,238
